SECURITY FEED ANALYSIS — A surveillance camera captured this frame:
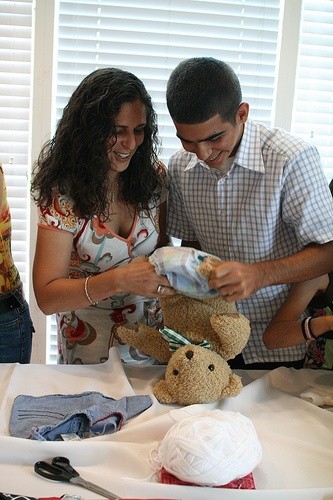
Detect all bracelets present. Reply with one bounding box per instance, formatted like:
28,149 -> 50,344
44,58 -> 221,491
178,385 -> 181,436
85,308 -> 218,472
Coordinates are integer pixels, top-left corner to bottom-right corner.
301,317 -> 316,343
85,276 -> 98,306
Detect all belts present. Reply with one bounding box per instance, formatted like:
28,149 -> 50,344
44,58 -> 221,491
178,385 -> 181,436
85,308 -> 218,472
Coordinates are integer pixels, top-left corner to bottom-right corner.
0,281 -> 27,314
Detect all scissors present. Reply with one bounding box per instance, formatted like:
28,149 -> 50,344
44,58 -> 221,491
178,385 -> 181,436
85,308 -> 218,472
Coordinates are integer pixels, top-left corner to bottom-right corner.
34,456 -> 118,499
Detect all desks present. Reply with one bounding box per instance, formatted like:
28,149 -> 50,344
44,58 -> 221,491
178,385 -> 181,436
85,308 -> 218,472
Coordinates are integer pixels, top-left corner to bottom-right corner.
0,347 -> 333,500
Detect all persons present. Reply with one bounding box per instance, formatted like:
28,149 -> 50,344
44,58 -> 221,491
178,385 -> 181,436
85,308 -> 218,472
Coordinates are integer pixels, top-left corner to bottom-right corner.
165,57 -> 333,370
31,67 -> 177,366
0,159 -> 36,364
262,178 -> 333,369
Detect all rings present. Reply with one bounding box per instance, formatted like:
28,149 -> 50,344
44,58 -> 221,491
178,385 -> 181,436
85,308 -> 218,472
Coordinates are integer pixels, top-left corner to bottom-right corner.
156,286 -> 164,294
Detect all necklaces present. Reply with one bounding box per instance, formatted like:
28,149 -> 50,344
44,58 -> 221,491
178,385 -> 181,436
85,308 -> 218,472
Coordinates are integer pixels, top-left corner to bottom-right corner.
112,184 -> 118,202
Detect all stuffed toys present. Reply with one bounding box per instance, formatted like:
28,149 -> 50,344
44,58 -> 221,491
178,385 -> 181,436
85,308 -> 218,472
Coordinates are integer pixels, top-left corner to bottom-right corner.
117,256 -> 251,405
